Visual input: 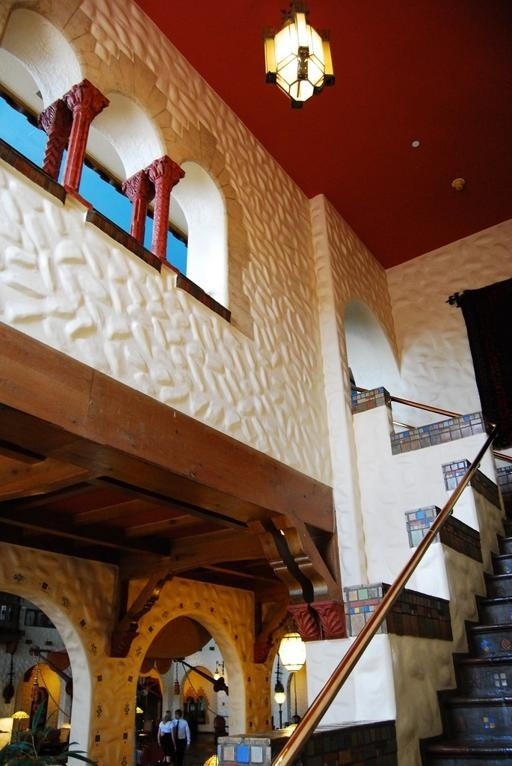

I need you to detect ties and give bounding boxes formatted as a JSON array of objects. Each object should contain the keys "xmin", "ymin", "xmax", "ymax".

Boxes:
[{"xmin": 175, "ymin": 720, "xmax": 180, "ymax": 739}]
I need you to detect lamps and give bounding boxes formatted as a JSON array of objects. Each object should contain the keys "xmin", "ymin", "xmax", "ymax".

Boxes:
[
  {"xmin": 260, "ymin": 2, "xmax": 337, "ymax": 111},
  {"xmin": 275, "ymin": 631, "xmax": 308, "ymax": 728}
]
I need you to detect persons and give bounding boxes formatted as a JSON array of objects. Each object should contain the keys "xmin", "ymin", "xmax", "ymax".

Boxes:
[
  {"xmin": 157, "ymin": 711, "xmax": 177, "ymax": 766},
  {"xmin": 170, "ymin": 710, "xmax": 193, "ymax": 766},
  {"xmin": 288, "ymin": 715, "xmax": 301, "ymax": 729}
]
